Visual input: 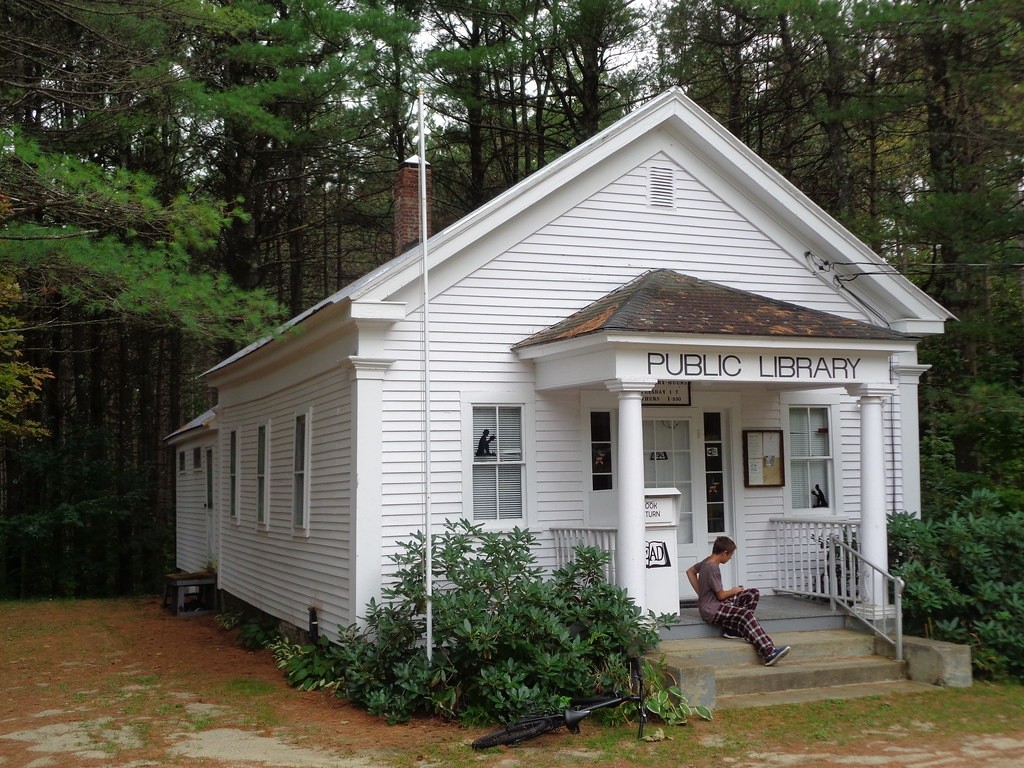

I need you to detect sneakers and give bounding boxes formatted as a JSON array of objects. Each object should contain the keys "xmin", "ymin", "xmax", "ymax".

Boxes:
[
  {"xmin": 764, "ymin": 645, "xmax": 791, "ymax": 665},
  {"xmin": 723, "ymin": 628, "xmax": 743, "ymax": 639}
]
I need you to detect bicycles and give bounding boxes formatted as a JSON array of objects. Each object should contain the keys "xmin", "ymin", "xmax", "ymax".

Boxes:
[{"xmin": 471, "ymin": 656, "xmax": 653, "ymax": 748}]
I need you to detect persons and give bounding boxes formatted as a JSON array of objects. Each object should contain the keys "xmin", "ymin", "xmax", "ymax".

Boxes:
[{"xmin": 685, "ymin": 535, "xmax": 791, "ymax": 667}]
